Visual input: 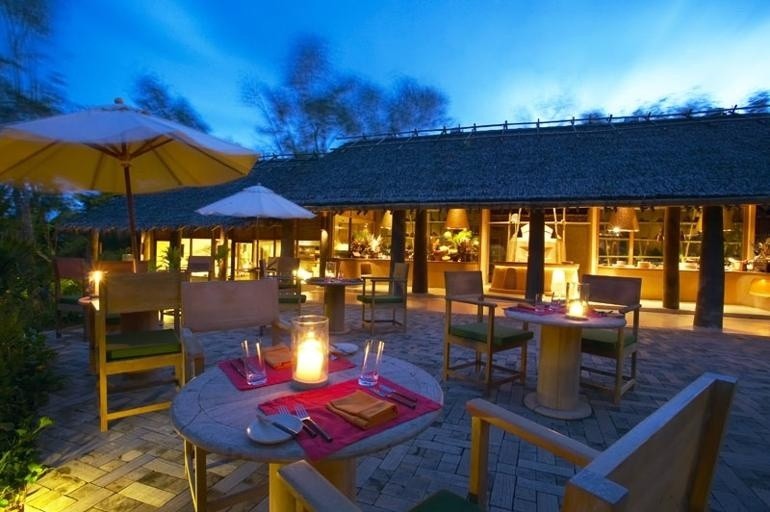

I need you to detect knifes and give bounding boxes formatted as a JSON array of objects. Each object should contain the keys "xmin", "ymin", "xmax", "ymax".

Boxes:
[
  {"xmin": 231, "ymin": 356, "xmax": 260, "ymax": 380},
  {"xmin": 378, "ymin": 383, "xmax": 418, "ymax": 402},
  {"xmin": 367, "ymin": 385, "xmax": 416, "ymax": 409},
  {"xmin": 256, "ymin": 413, "xmax": 298, "ymax": 437},
  {"xmin": 330, "ymin": 343, "xmax": 352, "ymax": 354}
]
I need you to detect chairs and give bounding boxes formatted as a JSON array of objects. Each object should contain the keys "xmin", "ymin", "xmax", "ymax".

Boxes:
[
  {"xmin": 356, "ymin": 262, "xmax": 410, "ymax": 337},
  {"xmin": 53, "ymin": 257, "xmax": 90, "ymax": 342},
  {"xmin": 275, "ymin": 372, "xmax": 738, "ymax": 512},
  {"xmin": 579, "ymin": 274, "xmax": 642, "ymax": 405},
  {"xmin": 267, "ymin": 257, "xmax": 301, "ymax": 316},
  {"xmin": 95, "ymin": 260, "xmax": 150, "ymax": 297},
  {"xmin": 443, "ymin": 271, "xmax": 533, "ymax": 397},
  {"xmin": 94, "ymin": 268, "xmax": 188, "ymax": 431},
  {"xmin": 186, "ymin": 256, "xmax": 216, "ymax": 283}
]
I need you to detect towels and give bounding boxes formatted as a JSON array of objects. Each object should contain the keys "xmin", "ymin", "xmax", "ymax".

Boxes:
[
  {"xmin": 262, "ymin": 342, "xmax": 291, "ymax": 370},
  {"xmin": 324, "ymin": 390, "xmax": 398, "ymax": 430}
]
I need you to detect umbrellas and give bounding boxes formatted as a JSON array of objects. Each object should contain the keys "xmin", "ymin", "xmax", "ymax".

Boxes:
[
  {"xmin": 193, "ymin": 182, "xmax": 316, "ymax": 268},
  {"xmin": 0, "ymin": 98, "xmax": 261, "ymax": 275}
]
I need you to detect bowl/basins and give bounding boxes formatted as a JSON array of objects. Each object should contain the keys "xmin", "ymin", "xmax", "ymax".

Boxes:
[{"xmin": 638, "ymin": 262, "xmax": 651, "ymax": 269}]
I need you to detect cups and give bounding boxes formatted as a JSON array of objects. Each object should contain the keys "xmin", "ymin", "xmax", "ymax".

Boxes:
[
  {"xmin": 566, "ymin": 282, "xmax": 590, "ymax": 319},
  {"xmin": 552, "ymin": 288, "xmax": 562, "ymax": 311},
  {"xmin": 290, "ymin": 315, "xmax": 329, "ymax": 390},
  {"xmin": 534, "ymin": 293, "xmax": 546, "ymax": 315},
  {"xmin": 358, "ymin": 338, "xmax": 385, "ymax": 386},
  {"xmin": 616, "ymin": 261, "xmax": 625, "ymax": 267},
  {"xmin": 240, "ymin": 337, "xmax": 267, "ymax": 385}
]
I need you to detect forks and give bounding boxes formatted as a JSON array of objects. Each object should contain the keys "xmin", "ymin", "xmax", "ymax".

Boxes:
[
  {"xmin": 278, "ymin": 405, "xmax": 316, "ymax": 438},
  {"xmin": 329, "ymin": 352, "xmax": 339, "ymax": 361},
  {"xmin": 294, "ymin": 403, "xmax": 332, "ymax": 441}
]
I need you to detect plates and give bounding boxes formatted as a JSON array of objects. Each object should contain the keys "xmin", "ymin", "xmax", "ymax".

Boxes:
[
  {"xmin": 245, "ymin": 414, "xmax": 304, "ymax": 445},
  {"xmin": 329, "ymin": 343, "xmax": 358, "ymax": 356}
]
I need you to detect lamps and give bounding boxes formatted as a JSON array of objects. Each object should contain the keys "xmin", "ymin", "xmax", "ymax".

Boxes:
[
  {"xmin": 378, "ymin": 209, "xmax": 393, "ymax": 229},
  {"xmin": 607, "ymin": 206, "xmax": 641, "ymax": 233},
  {"xmin": 442, "ymin": 209, "xmax": 470, "ymax": 229}
]
